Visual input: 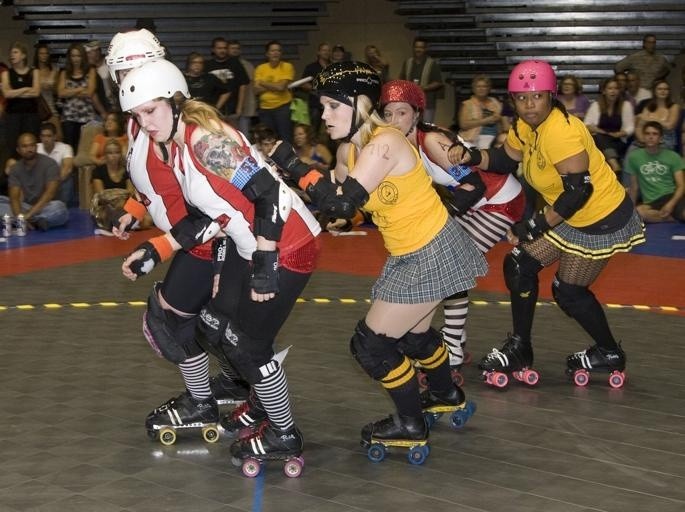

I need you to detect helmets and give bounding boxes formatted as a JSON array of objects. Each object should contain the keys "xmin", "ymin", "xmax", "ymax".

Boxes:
[
  {"xmin": 507, "ymin": 61, "xmax": 558, "ymax": 93},
  {"xmin": 313, "ymin": 60, "xmax": 382, "ymax": 108},
  {"xmin": 104, "ymin": 27, "xmax": 167, "ymax": 85},
  {"xmin": 381, "ymin": 79, "xmax": 426, "ymax": 109},
  {"xmin": 118, "ymin": 58, "xmax": 192, "ymax": 114}
]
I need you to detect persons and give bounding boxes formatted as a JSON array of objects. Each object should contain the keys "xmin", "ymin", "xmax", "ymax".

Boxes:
[
  {"xmin": 2, "ymin": 15, "xmax": 685, "ymax": 240},
  {"xmin": 120, "ymin": 59, "xmax": 323, "ymax": 478},
  {"xmin": 446, "ymin": 60, "xmax": 649, "ymax": 389},
  {"xmin": 104, "ymin": 28, "xmax": 251, "ymax": 447},
  {"xmin": 380, "ymin": 80, "xmax": 528, "ymax": 393},
  {"xmin": 267, "ymin": 59, "xmax": 489, "ymax": 467}
]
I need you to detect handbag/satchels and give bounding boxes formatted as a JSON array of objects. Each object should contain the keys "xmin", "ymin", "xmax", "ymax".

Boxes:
[{"xmin": 36, "ymin": 95, "xmax": 52, "ymax": 121}]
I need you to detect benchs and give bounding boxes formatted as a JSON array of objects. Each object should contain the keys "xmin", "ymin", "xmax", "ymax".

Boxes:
[{"xmin": 393, "ymin": 0, "xmax": 685, "ymax": 133}]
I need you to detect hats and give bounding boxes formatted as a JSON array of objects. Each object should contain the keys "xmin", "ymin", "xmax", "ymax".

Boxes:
[{"xmin": 333, "ymin": 44, "xmax": 344, "ymax": 52}]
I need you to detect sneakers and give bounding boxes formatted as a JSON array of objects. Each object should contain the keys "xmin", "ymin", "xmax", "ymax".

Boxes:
[{"xmin": 30, "ymin": 219, "xmax": 48, "ymax": 232}]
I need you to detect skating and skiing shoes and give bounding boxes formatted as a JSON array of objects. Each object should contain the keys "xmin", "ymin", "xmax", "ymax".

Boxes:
[
  {"xmin": 419, "ymin": 385, "xmax": 476, "ymax": 431},
  {"xmin": 144, "ymin": 370, "xmax": 304, "ymax": 478},
  {"xmin": 416, "ymin": 340, "xmax": 626, "ymax": 388},
  {"xmin": 360, "ymin": 412, "xmax": 430, "ymax": 464}
]
